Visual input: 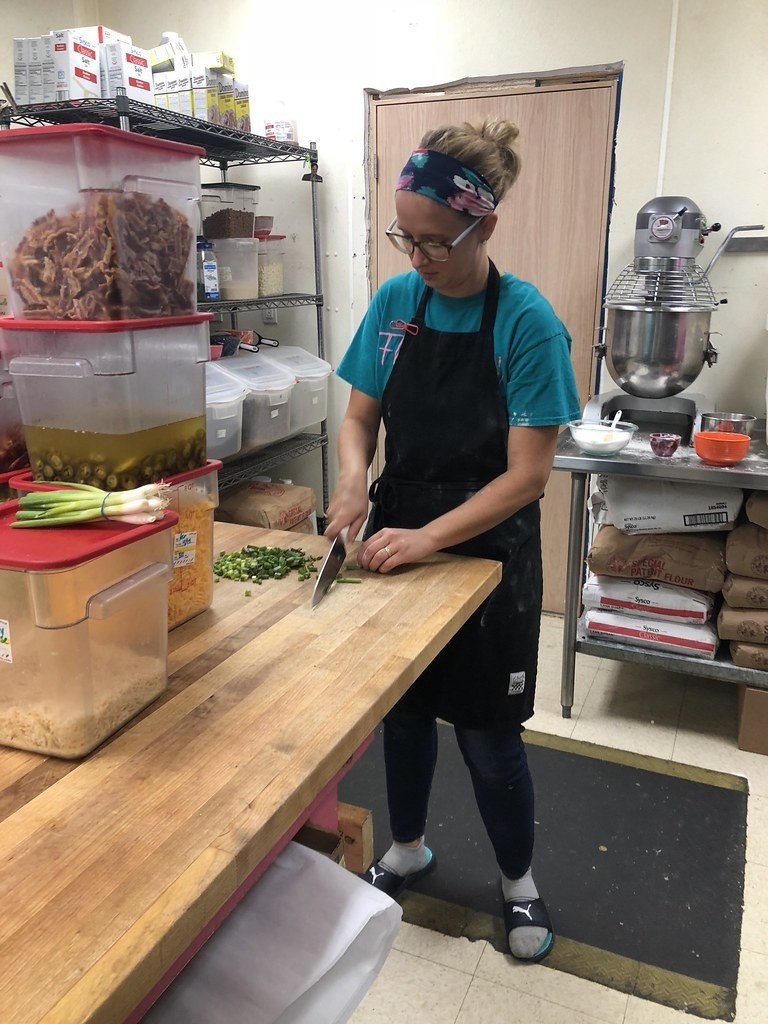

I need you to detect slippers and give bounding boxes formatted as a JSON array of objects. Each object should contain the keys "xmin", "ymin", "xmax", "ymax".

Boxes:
[
  {"xmin": 499, "ymin": 877, "xmax": 554, "ymax": 962},
  {"xmin": 364, "ymin": 856, "xmax": 437, "ymax": 900}
]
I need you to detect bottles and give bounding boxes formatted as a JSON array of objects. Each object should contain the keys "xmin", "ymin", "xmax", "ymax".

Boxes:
[{"xmin": 196, "ymin": 243, "xmax": 221, "ymax": 301}]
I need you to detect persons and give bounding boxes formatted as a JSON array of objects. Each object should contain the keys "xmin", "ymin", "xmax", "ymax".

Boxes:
[{"xmin": 323, "ymin": 118, "xmax": 583, "ymax": 964}]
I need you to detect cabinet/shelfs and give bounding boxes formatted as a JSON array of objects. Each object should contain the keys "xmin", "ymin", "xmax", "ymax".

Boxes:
[
  {"xmin": 550, "ymin": 416, "xmax": 768, "ymax": 720},
  {"xmin": 0, "ymin": 86, "xmax": 331, "ymax": 536}
]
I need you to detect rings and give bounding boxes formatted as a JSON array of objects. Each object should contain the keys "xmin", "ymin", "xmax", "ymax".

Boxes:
[{"xmin": 384, "ymin": 547, "xmax": 392, "ymax": 558}]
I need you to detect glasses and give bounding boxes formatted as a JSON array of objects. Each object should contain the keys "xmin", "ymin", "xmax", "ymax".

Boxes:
[{"xmin": 386, "ymin": 217, "xmax": 482, "ymax": 264}]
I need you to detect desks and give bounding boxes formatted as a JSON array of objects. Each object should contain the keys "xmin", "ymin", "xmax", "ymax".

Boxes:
[{"xmin": 0, "ymin": 522, "xmax": 502, "ymax": 1023}]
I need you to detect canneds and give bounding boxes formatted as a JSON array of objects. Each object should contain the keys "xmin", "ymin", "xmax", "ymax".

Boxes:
[{"xmin": 196, "ymin": 242, "xmax": 221, "ymax": 303}]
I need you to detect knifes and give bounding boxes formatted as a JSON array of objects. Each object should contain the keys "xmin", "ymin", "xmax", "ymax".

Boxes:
[{"xmin": 310, "ymin": 525, "xmax": 349, "ymax": 607}]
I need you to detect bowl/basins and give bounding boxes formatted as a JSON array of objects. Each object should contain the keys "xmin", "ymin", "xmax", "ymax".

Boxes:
[
  {"xmin": 694, "ymin": 431, "xmax": 751, "ymax": 466},
  {"xmin": 649, "ymin": 433, "xmax": 682, "ymax": 456},
  {"xmin": 701, "ymin": 413, "xmax": 757, "ymax": 435},
  {"xmin": 567, "ymin": 420, "xmax": 638, "ymax": 456}
]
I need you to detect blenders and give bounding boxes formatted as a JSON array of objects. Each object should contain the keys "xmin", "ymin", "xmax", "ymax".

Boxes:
[{"xmin": 602, "ymin": 195, "xmax": 717, "ymax": 441}]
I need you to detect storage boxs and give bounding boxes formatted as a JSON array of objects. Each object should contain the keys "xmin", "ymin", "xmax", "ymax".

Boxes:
[
  {"xmin": 737, "ymin": 685, "xmax": 768, "ymax": 755},
  {"xmin": 198, "ymin": 183, "xmax": 261, "ymax": 241},
  {"xmin": 202, "ymin": 238, "xmax": 260, "ymax": 301},
  {"xmin": 8, "ymin": 458, "xmax": 223, "ymax": 634},
  {"xmin": 0, "ymin": 314, "xmax": 216, "ymax": 494},
  {"xmin": 254, "ymin": 235, "xmax": 286, "ymax": 299},
  {"xmin": 12, "ymin": 24, "xmax": 251, "ymax": 135},
  {"xmin": 1, "ymin": 123, "xmax": 207, "ymax": 321},
  {"xmin": 203, "ymin": 362, "xmax": 252, "ymax": 459},
  {"xmin": 1, "ymin": 497, "xmax": 180, "ymax": 762},
  {"xmin": 258, "ymin": 344, "xmax": 332, "ymax": 429},
  {"xmin": 337, "ymin": 801, "xmax": 374, "ymax": 875},
  {"xmin": 214, "ymin": 352, "xmax": 300, "ymax": 449}
]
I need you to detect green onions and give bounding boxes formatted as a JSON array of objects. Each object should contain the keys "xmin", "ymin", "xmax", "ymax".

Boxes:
[
  {"xmin": 213, "ymin": 544, "xmax": 364, "ymax": 596},
  {"xmin": 9, "ymin": 480, "xmax": 172, "ymax": 524}
]
